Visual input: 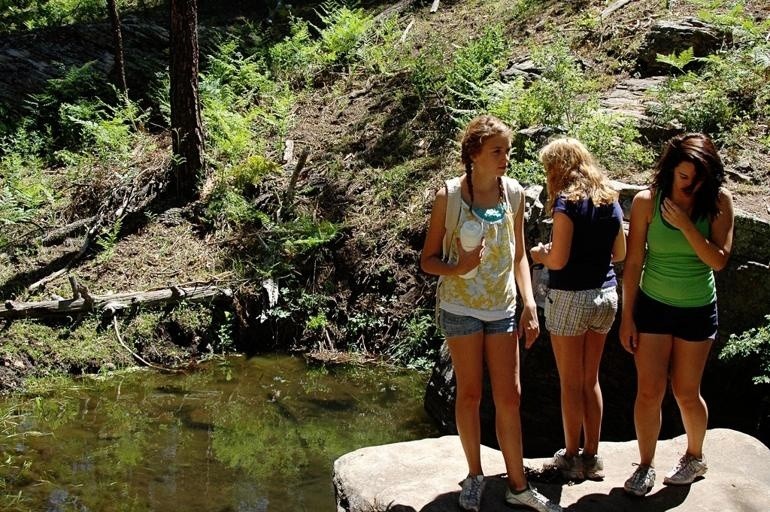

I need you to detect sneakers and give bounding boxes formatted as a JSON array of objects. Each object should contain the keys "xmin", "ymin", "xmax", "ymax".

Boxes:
[
  {"xmin": 623, "ymin": 463, "xmax": 658, "ymax": 499},
  {"xmin": 661, "ymin": 452, "xmax": 709, "ymax": 488},
  {"xmin": 457, "ymin": 472, "xmax": 488, "ymax": 509},
  {"xmin": 502, "ymin": 481, "xmax": 563, "ymax": 512},
  {"xmin": 554, "ymin": 445, "xmax": 607, "ymax": 485}
]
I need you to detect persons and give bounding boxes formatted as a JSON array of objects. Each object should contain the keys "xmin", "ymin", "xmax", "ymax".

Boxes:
[
  {"xmin": 616, "ymin": 133, "xmax": 736, "ymax": 499},
  {"xmin": 419, "ymin": 113, "xmax": 569, "ymax": 512},
  {"xmin": 527, "ymin": 136, "xmax": 629, "ymax": 486}
]
